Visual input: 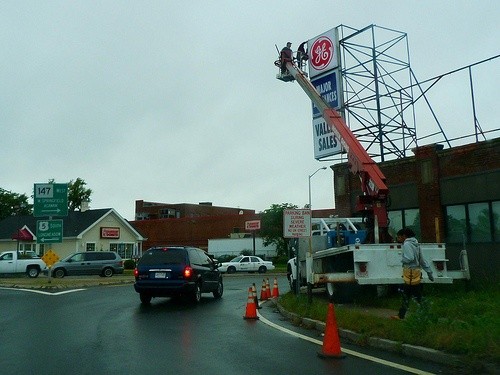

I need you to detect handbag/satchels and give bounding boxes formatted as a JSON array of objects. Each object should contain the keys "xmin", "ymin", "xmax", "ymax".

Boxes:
[{"xmin": 403, "ymin": 267, "xmax": 422, "ymax": 286}]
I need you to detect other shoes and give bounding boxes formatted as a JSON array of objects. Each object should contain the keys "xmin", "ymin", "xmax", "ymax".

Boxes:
[{"xmin": 390, "ymin": 315, "xmax": 400, "ymax": 319}]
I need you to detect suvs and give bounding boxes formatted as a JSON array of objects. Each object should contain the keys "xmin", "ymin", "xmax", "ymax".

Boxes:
[{"xmin": 134, "ymin": 246, "xmax": 223, "ymax": 305}]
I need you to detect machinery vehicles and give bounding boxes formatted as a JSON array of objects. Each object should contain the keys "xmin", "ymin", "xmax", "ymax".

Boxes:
[{"xmin": 275, "ymin": 44, "xmax": 471, "ymax": 297}]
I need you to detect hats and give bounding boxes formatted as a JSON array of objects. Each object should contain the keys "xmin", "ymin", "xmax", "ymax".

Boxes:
[{"xmin": 287, "ymin": 42, "xmax": 292, "ymax": 44}]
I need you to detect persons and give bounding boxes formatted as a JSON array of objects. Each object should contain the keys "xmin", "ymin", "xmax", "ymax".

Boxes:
[
  {"xmin": 279, "ymin": 42, "xmax": 292, "ymax": 75},
  {"xmin": 296, "ymin": 41, "xmax": 308, "ymax": 68},
  {"xmin": 390, "ymin": 228, "xmax": 433, "ymax": 321}
]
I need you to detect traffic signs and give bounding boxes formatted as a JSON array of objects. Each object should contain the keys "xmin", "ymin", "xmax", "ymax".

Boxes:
[
  {"xmin": 34, "ymin": 183, "xmax": 68, "ymax": 218},
  {"xmin": 36, "ymin": 219, "xmax": 63, "ymax": 244}
]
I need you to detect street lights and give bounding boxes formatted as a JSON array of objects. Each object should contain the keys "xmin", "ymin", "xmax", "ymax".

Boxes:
[
  {"xmin": 306, "ymin": 166, "xmax": 327, "ymax": 303},
  {"xmin": 14, "ymin": 205, "xmax": 21, "ymax": 250}
]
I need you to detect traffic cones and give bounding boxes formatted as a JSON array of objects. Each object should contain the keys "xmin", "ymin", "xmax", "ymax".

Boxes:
[
  {"xmin": 315, "ymin": 302, "xmax": 347, "ymax": 359},
  {"xmin": 242, "ymin": 283, "xmax": 262, "ymax": 320},
  {"xmin": 258, "ymin": 277, "xmax": 273, "ymax": 301},
  {"xmin": 270, "ymin": 277, "xmax": 279, "ymax": 299}
]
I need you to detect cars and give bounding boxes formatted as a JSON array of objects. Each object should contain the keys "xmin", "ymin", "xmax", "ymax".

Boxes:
[
  {"xmin": 213, "ymin": 255, "xmax": 275, "ymax": 274},
  {"xmin": 0, "ymin": 250, "xmax": 47, "ymax": 278}
]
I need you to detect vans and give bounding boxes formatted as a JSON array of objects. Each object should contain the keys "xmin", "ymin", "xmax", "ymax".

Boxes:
[{"xmin": 44, "ymin": 251, "xmax": 125, "ymax": 279}]
